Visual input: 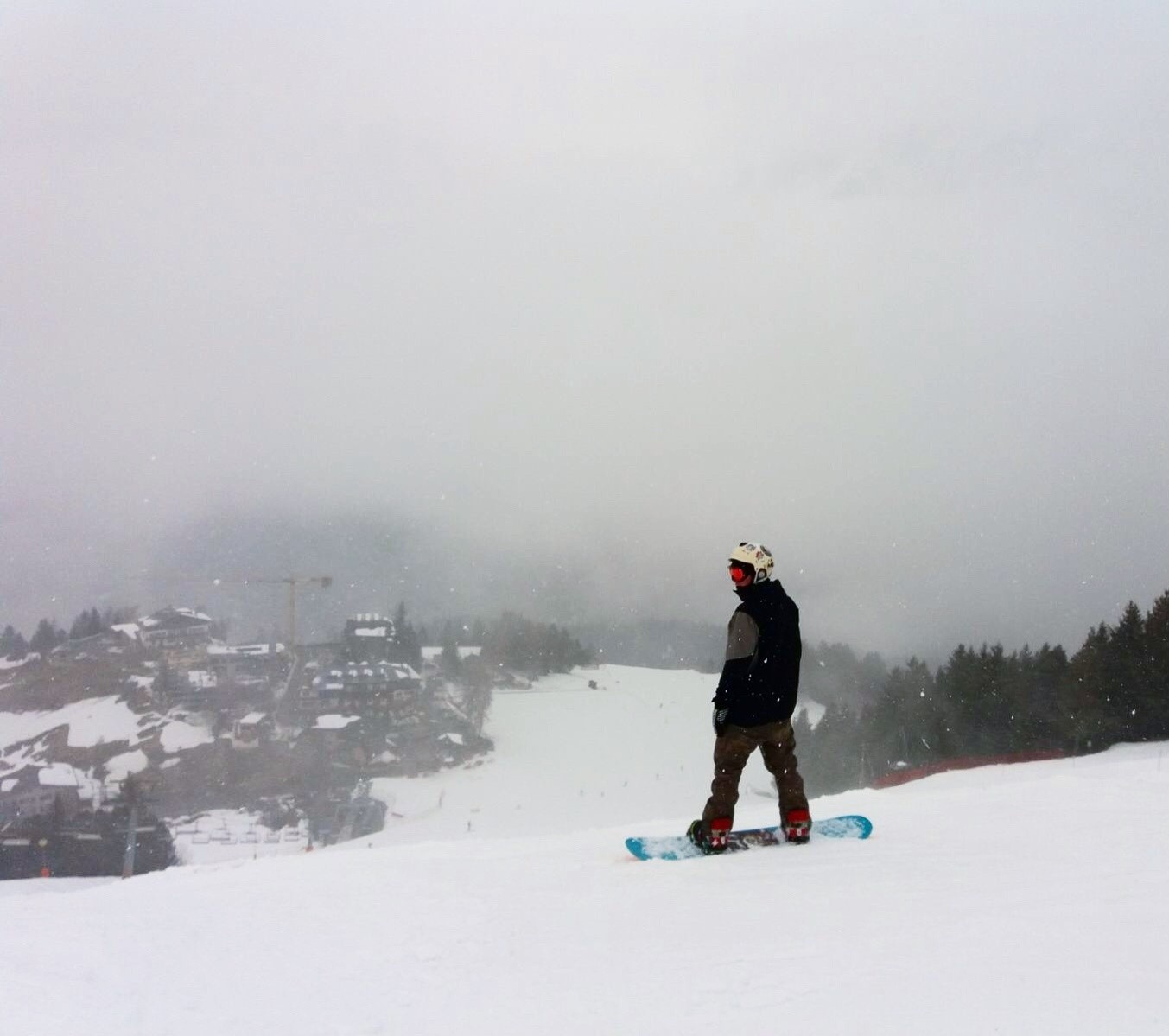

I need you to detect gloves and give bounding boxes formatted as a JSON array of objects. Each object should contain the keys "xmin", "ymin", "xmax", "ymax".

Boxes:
[{"xmin": 710, "ymin": 691, "xmax": 732, "ymax": 738}]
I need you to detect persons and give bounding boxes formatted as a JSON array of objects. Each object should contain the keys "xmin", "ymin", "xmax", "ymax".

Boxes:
[{"xmin": 687, "ymin": 542, "xmax": 812, "ymax": 856}]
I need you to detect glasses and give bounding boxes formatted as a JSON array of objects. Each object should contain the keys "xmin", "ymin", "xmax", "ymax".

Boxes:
[{"xmin": 728, "ymin": 565, "xmax": 755, "ymax": 582}]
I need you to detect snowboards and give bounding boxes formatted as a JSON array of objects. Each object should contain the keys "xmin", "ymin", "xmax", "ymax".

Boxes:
[{"xmin": 625, "ymin": 815, "xmax": 873, "ymax": 861}]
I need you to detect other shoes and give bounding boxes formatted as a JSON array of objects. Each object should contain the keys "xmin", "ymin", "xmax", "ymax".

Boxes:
[
  {"xmin": 786, "ymin": 828, "xmax": 813, "ymax": 846},
  {"xmin": 690, "ymin": 821, "xmax": 729, "ymax": 857}
]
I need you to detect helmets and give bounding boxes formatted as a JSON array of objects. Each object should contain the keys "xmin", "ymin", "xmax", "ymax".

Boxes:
[{"xmin": 726, "ymin": 542, "xmax": 776, "ymax": 585}]
